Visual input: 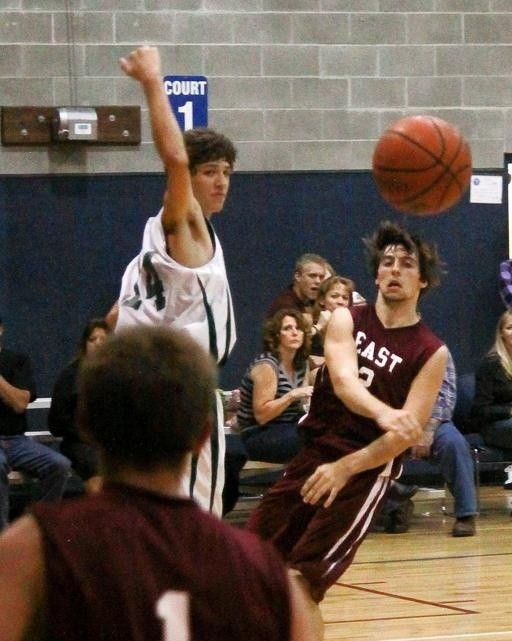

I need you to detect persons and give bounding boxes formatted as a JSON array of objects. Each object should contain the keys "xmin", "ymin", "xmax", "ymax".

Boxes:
[
  {"xmin": 240, "ymin": 219, "xmax": 444, "ymax": 602},
  {"xmin": 0, "ymin": 318, "xmax": 113, "ymax": 507},
  {"xmin": 104, "ymin": 38, "xmax": 239, "ymax": 518},
  {"xmin": 1, "ymin": 322, "xmax": 327, "ymax": 641},
  {"xmin": 219, "ymin": 249, "xmax": 511, "ymax": 542}
]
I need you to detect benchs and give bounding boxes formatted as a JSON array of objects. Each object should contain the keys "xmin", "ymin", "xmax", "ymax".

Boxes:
[{"xmin": 8, "ymin": 392, "xmax": 283, "ymax": 487}]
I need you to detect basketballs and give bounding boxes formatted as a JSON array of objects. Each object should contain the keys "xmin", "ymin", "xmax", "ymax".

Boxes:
[{"xmin": 371, "ymin": 114, "xmax": 473, "ymax": 219}]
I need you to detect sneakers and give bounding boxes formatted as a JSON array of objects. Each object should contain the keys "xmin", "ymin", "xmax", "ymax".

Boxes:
[
  {"xmin": 453, "ymin": 516, "xmax": 474, "ymax": 536},
  {"xmin": 387, "ymin": 500, "xmax": 413, "ymax": 533}
]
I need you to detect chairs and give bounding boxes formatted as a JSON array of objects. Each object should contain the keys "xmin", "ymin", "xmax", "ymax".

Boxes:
[{"xmin": 442, "ymin": 373, "xmax": 512, "ymax": 517}]
